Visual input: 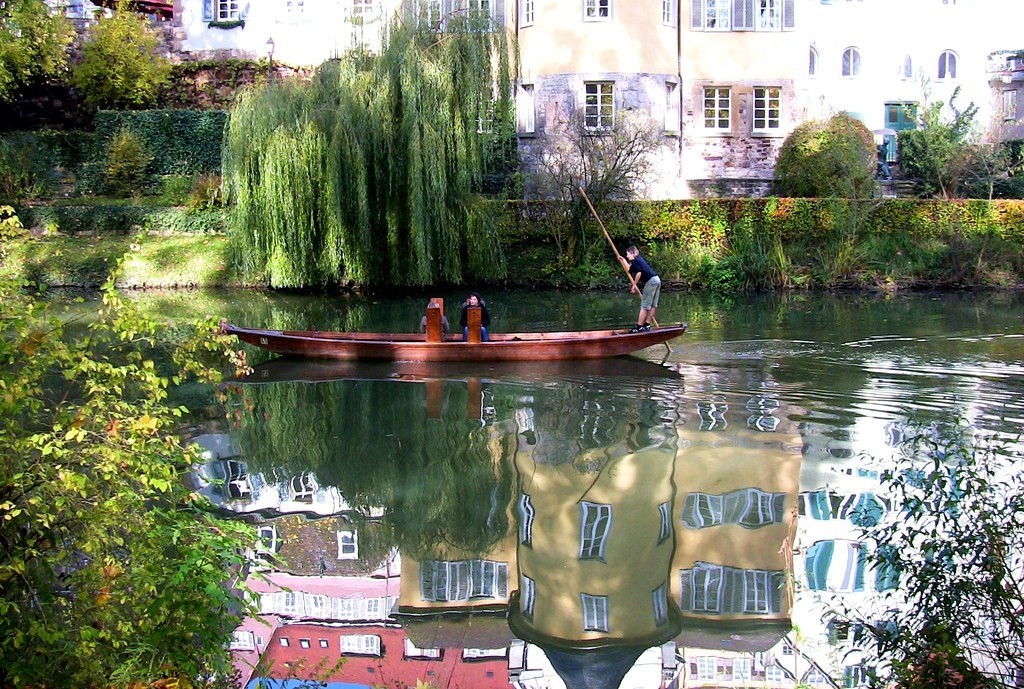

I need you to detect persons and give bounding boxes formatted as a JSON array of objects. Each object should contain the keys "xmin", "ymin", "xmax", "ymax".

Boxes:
[
  {"xmin": 420, "ymin": 302, "xmax": 449, "ymax": 343},
  {"xmin": 876, "ymin": 138, "xmax": 893, "ymax": 180},
  {"xmin": 460, "ymin": 292, "xmax": 491, "ymax": 342},
  {"xmin": 618, "ymin": 246, "xmax": 661, "ymax": 335}
]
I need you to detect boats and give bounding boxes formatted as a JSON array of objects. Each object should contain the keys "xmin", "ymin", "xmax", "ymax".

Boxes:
[
  {"xmin": 221, "ymin": 361, "xmax": 685, "ymax": 420},
  {"xmin": 206, "ymin": 297, "xmax": 688, "ymax": 361}
]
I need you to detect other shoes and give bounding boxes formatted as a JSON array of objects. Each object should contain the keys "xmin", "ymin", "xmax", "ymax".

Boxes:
[
  {"xmin": 643, "ymin": 324, "xmax": 650, "ymax": 331},
  {"xmin": 629, "ymin": 327, "xmax": 643, "ymax": 333}
]
